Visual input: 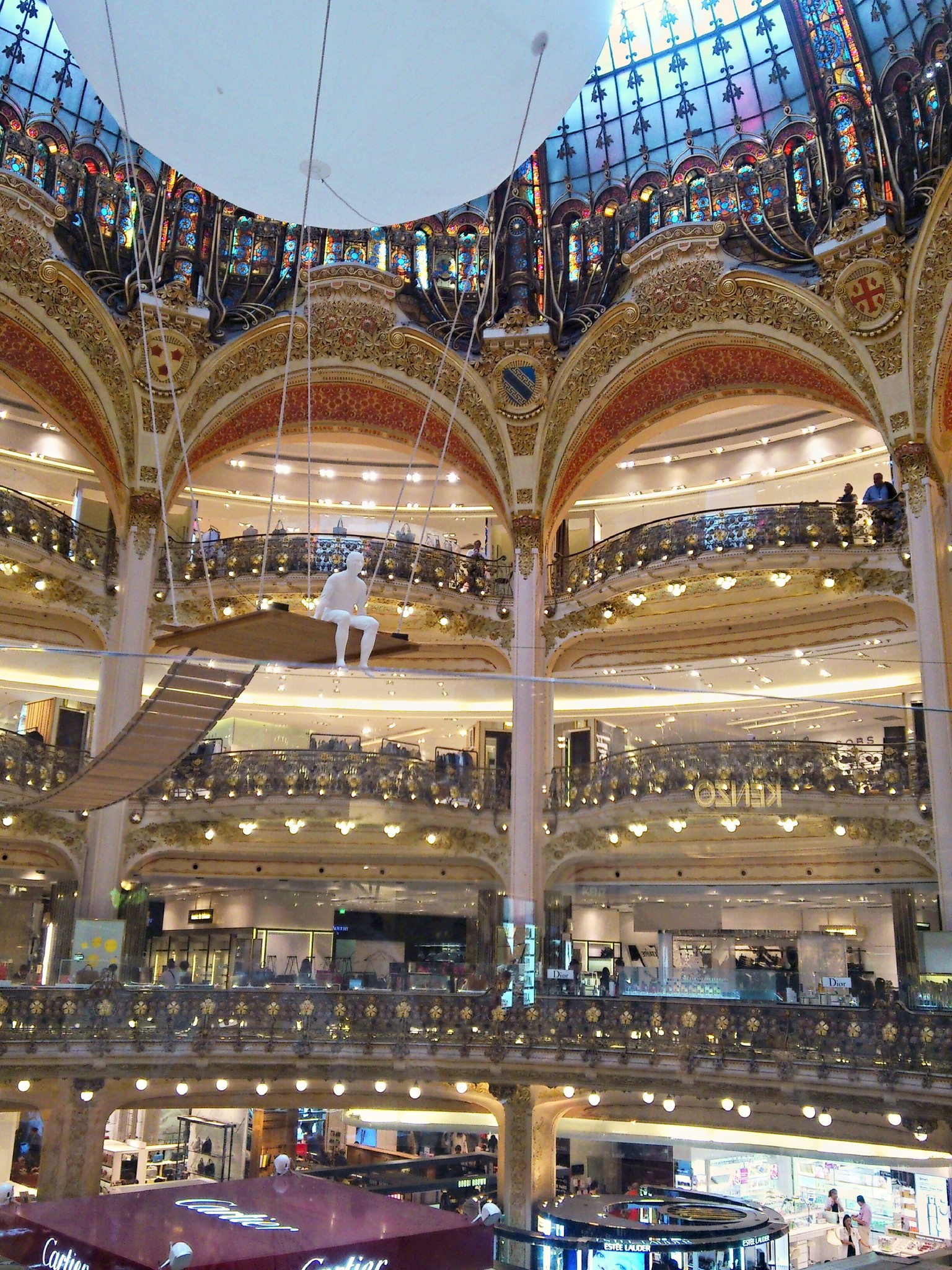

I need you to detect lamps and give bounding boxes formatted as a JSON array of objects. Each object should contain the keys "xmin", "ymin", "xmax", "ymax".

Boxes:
[{"xmin": 0, "ymin": 539, "xmax": 927, "ymax": 1141}]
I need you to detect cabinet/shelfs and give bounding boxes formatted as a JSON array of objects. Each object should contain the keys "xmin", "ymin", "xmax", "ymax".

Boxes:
[
  {"xmin": 573, "ymin": 940, "xmax": 622, "ymax": 974},
  {"xmin": 101, "ymin": 1115, "xmax": 236, "ymax": 1186},
  {"xmin": 156, "ymin": 965, "xmax": 228, "ymax": 977},
  {"xmin": 705, "ymin": 1155, "xmax": 919, "ymax": 1270}
]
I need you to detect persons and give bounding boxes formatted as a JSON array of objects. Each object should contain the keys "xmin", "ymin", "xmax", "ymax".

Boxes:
[
  {"xmin": 592, "ymin": 1249, "xmax": 644, "ymax": 1270},
  {"xmin": 837, "ymin": 473, "xmax": 899, "ymax": 545},
  {"xmin": 13, "ymin": 965, "xmax": 29, "ymax": 981},
  {"xmin": 466, "ymin": 540, "xmax": 487, "ymax": 594},
  {"xmin": 824, "ymin": 1189, "xmax": 872, "ymax": 1258},
  {"xmin": 311, "ymin": 552, "xmax": 380, "ymax": 677},
  {"xmin": 333, "ymin": 1148, "xmax": 347, "ymax": 1183},
  {"xmin": 18, "ymin": 1128, "xmax": 41, "ymax": 1174},
  {"xmin": 465, "ymin": 964, "xmax": 487, "ymax": 991},
  {"xmin": 159, "ymin": 958, "xmax": 192, "ymax": 987},
  {"xmin": 588, "ymin": 1180, "xmax": 641, "ymax": 1221},
  {"xmin": 565, "ymin": 958, "xmax": 629, "ymax": 998}
]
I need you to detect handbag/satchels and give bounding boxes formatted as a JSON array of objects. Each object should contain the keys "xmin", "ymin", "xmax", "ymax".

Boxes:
[
  {"xmin": 76, "ymin": 965, "xmax": 98, "ymax": 984},
  {"xmin": 146, "ymin": 1167, "xmax": 158, "ymax": 1179},
  {"xmin": 250, "ymin": 958, "xmax": 342, "ymax": 986},
  {"xmin": 202, "ymin": 1137, "xmax": 213, "ymax": 1153},
  {"xmin": 197, "ymin": 1158, "xmax": 205, "ymax": 1174},
  {"xmin": 272, "ymin": 521, "xmax": 287, "ymax": 539},
  {"xmin": 172, "ymin": 1148, "xmax": 184, "ymax": 1160},
  {"xmin": 163, "ymin": 1168, "xmax": 173, "ymax": 1177},
  {"xmin": 152, "ymin": 1151, "xmax": 164, "ymax": 1163},
  {"xmin": 395, "ymin": 523, "xmax": 415, "ymax": 547},
  {"xmin": 190, "ymin": 1137, "xmax": 201, "ymax": 1151},
  {"xmin": 204, "ymin": 1159, "xmax": 215, "ymax": 1175},
  {"xmin": 333, "ymin": 519, "xmax": 347, "ymax": 538}
]
